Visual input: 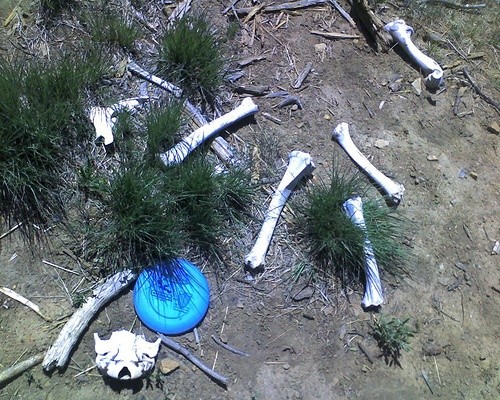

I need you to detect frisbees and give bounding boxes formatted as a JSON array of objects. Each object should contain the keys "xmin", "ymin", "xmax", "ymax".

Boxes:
[{"xmin": 133, "ymin": 258, "xmax": 210, "ymax": 336}]
